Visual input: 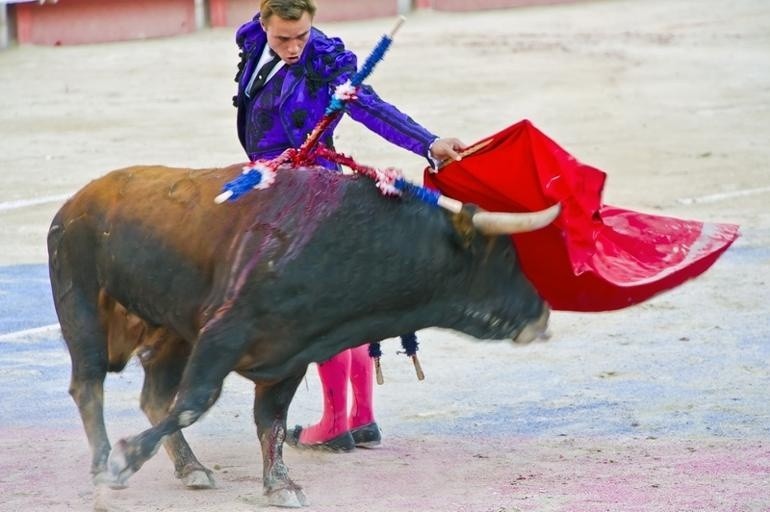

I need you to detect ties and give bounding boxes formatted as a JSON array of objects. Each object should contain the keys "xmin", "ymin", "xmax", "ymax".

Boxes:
[{"xmin": 250, "ymin": 47, "xmax": 283, "ymax": 98}]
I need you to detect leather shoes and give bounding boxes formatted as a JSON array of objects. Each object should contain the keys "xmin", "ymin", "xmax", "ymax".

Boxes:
[{"xmin": 283, "ymin": 422, "xmax": 382, "ymax": 454}]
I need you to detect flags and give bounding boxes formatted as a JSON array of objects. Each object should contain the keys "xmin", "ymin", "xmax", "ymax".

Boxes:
[{"xmin": 427, "ymin": 120, "xmax": 742, "ymax": 314}]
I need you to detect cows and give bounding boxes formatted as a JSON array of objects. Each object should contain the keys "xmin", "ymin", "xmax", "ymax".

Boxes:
[{"xmin": 45, "ymin": 160, "xmax": 561, "ymax": 509}]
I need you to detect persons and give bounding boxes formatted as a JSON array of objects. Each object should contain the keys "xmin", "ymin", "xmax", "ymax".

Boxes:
[{"xmin": 235, "ymin": 0, "xmax": 466, "ymax": 455}]
